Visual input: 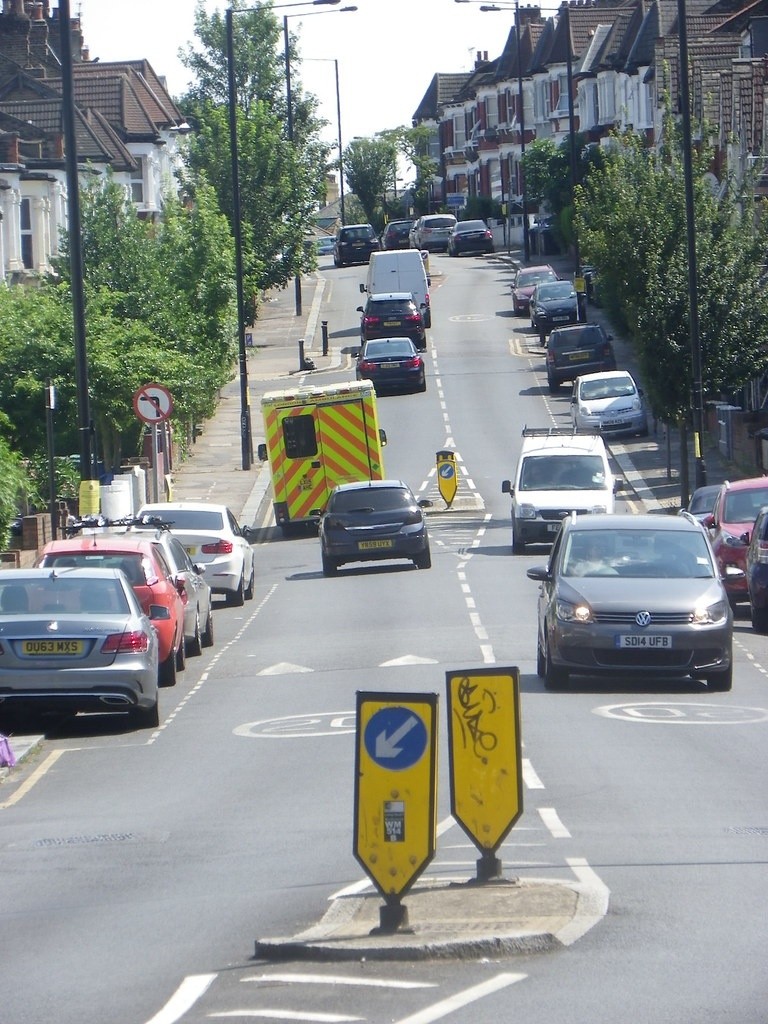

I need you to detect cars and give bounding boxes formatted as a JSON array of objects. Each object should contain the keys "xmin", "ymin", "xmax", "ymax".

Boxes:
[
  {"xmin": 447, "ymin": 220, "xmax": 495, "ymax": 257},
  {"xmin": 409, "ymin": 219, "xmax": 419, "ymax": 249},
  {"xmin": 529, "ymin": 281, "xmax": 577, "ymax": 333},
  {"xmin": 413, "ymin": 215, "xmax": 457, "ymax": 252},
  {"xmin": 351, "ymin": 337, "xmax": 428, "ymax": 393},
  {"xmin": 677, "ymin": 487, "xmax": 723, "ymax": 529},
  {"xmin": 141, "ymin": 503, "xmax": 257, "ymax": 608},
  {"xmin": 275, "ymin": 246, "xmax": 289, "ymax": 260},
  {"xmin": 31, "ymin": 536, "xmax": 189, "ymax": 687},
  {"xmin": 380, "ymin": 219, "xmax": 413, "ymax": 251},
  {"xmin": 308, "ymin": 480, "xmax": 432, "ymax": 578},
  {"xmin": 572, "ymin": 371, "xmax": 649, "ymax": 439},
  {"xmin": 0, "ymin": 567, "xmax": 171, "ymax": 730},
  {"xmin": 316, "ymin": 236, "xmax": 336, "ymax": 256},
  {"xmin": 741, "ymin": 507, "xmax": 768, "ymax": 634}
]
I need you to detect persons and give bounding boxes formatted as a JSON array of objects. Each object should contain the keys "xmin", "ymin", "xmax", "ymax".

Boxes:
[
  {"xmin": 528, "ymin": 467, "xmax": 552, "ymax": 488},
  {"xmin": 574, "ymin": 546, "xmax": 608, "ymax": 576}
]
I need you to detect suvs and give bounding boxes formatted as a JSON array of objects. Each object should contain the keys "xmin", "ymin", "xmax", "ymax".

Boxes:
[
  {"xmin": 356, "ymin": 292, "xmax": 429, "ymax": 353},
  {"xmin": 543, "ymin": 322, "xmax": 619, "ymax": 393},
  {"xmin": 703, "ymin": 473, "xmax": 767, "ymax": 600},
  {"xmin": 526, "ymin": 510, "xmax": 746, "ymax": 691},
  {"xmin": 61, "ymin": 513, "xmax": 213, "ymax": 658},
  {"xmin": 501, "ymin": 426, "xmax": 623, "ymax": 553},
  {"xmin": 330, "ymin": 223, "xmax": 380, "ymax": 268},
  {"xmin": 511, "ymin": 264, "xmax": 564, "ymax": 315}
]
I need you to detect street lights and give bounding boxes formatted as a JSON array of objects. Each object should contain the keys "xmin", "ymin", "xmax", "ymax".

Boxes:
[
  {"xmin": 453, "ymin": 0, "xmax": 531, "ymax": 264},
  {"xmin": 291, "ymin": 57, "xmax": 346, "ymax": 228},
  {"xmin": 480, "ymin": 5, "xmax": 577, "ymax": 190},
  {"xmin": 285, "ymin": 5, "xmax": 358, "ymax": 321},
  {"xmin": 225, "ymin": 0, "xmax": 342, "ymax": 474}
]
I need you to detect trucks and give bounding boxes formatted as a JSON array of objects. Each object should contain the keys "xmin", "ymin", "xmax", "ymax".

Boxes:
[
  {"xmin": 257, "ymin": 379, "xmax": 387, "ymax": 535},
  {"xmin": 358, "ymin": 250, "xmax": 433, "ymax": 330}
]
